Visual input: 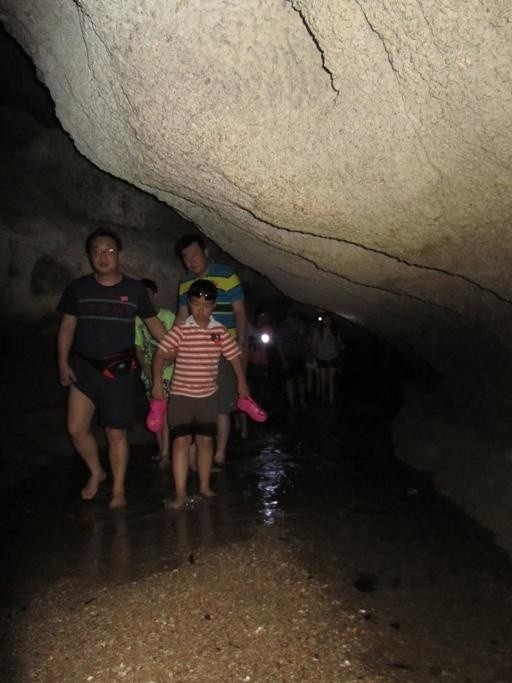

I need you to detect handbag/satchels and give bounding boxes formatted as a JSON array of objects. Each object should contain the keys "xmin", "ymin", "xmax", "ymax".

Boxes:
[{"xmin": 74, "ymin": 350, "xmax": 140, "ymax": 384}]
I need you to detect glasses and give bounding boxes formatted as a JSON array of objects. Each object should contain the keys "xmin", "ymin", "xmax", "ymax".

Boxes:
[
  {"xmin": 92, "ymin": 248, "xmax": 115, "ymax": 256},
  {"xmin": 190, "ymin": 290, "xmax": 215, "ymax": 300}
]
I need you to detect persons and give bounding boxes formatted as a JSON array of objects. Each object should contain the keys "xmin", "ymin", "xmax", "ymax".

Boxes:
[
  {"xmin": 57, "ymin": 228, "xmax": 168, "ymax": 506},
  {"xmin": 251, "ymin": 311, "xmax": 339, "ymax": 407},
  {"xmin": 153, "ymin": 280, "xmax": 251, "ymax": 507},
  {"xmin": 134, "ymin": 278, "xmax": 176, "ymax": 461},
  {"xmin": 171, "ymin": 231, "xmax": 247, "ymax": 464}
]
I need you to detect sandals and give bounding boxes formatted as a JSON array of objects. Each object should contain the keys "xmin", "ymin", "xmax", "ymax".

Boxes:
[
  {"xmin": 146, "ymin": 399, "xmax": 167, "ymax": 432},
  {"xmin": 237, "ymin": 397, "xmax": 268, "ymax": 423}
]
